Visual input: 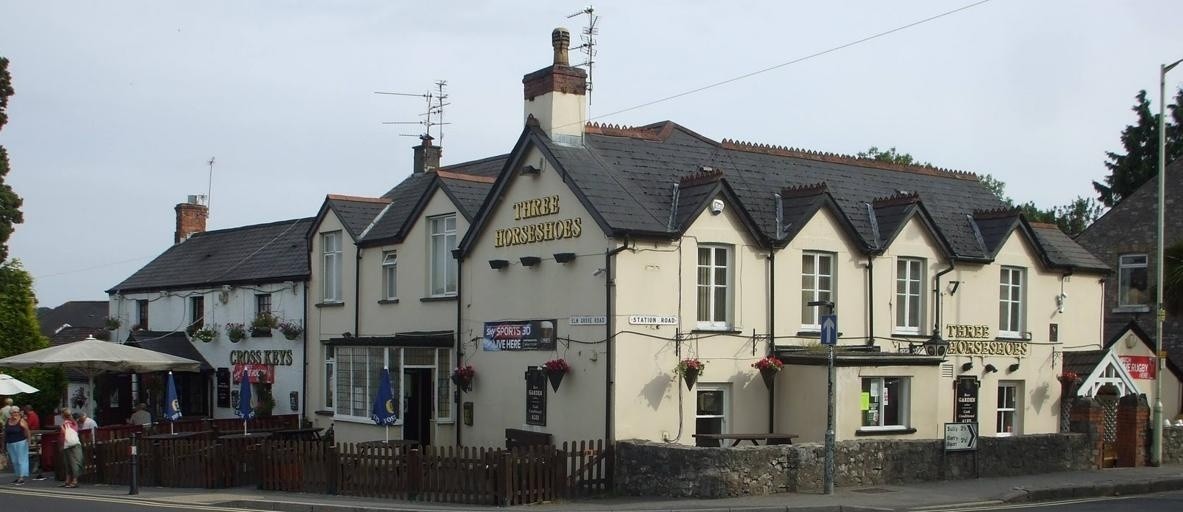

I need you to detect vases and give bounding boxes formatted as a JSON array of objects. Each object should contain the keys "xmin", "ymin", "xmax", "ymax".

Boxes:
[
  {"xmin": 547, "ymin": 369, "xmax": 566, "ymax": 393},
  {"xmin": 760, "ymin": 367, "xmax": 781, "ymax": 390},
  {"xmin": 682, "ymin": 368, "xmax": 698, "ymax": 391}
]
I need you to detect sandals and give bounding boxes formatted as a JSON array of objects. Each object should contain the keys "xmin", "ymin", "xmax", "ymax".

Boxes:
[{"xmin": 58, "ymin": 481, "xmax": 78, "ymax": 488}]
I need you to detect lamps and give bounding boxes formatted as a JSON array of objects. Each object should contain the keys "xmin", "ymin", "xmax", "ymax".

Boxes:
[
  {"xmin": 908, "ymin": 329, "xmax": 949, "ymax": 362},
  {"xmin": 521, "ymin": 165, "xmax": 541, "ymax": 177}
]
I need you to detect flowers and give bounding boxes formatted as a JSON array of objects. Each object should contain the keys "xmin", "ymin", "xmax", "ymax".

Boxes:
[
  {"xmin": 542, "ymin": 359, "xmax": 568, "ymax": 371},
  {"xmin": 751, "ymin": 354, "xmax": 784, "ymax": 371},
  {"xmin": 672, "ymin": 358, "xmax": 704, "ymax": 377}
]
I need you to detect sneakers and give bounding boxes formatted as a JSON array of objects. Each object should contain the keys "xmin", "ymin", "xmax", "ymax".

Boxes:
[{"xmin": 10, "ymin": 479, "xmax": 24, "ymax": 485}]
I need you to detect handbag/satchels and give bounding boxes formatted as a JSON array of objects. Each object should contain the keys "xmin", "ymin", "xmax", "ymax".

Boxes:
[{"xmin": 64, "ymin": 425, "xmax": 80, "ymax": 449}]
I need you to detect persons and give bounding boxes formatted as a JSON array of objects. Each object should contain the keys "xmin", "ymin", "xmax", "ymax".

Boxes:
[
  {"xmin": 72, "ymin": 411, "xmax": 98, "ymax": 429},
  {"xmin": 0, "ymin": 397, "xmax": 14, "ymax": 432},
  {"xmin": 2, "ymin": 405, "xmax": 31, "ymax": 486},
  {"xmin": 56, "ymin": 407, "xmax": 83, "ymax": 488},
  {"xmin": 22, "ymin": 404, "xmax": 40, "ymax": 430},
  {"xmin": 127, "ymin": 402, "xmax": 151, "ymax": 426}
]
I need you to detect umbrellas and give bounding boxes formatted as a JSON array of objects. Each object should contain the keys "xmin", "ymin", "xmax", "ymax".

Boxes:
[
  {"xmin": 0, "ymin": 332, "xmax": 203, "ymax": 443},
  {"xmin": 0, "ymin": 373, "xmax": 40, "ymax": 399},
  {"xmin": 162, "ymin": 370, "xmax": 183, "ymax": 435},
  {"xmin": 237, "ymin": 366, "xmax": 256, "ymax": 435},
  {"xmin": 368, "ymin": 366, "xmax": 397, "ymax": 443}
]
